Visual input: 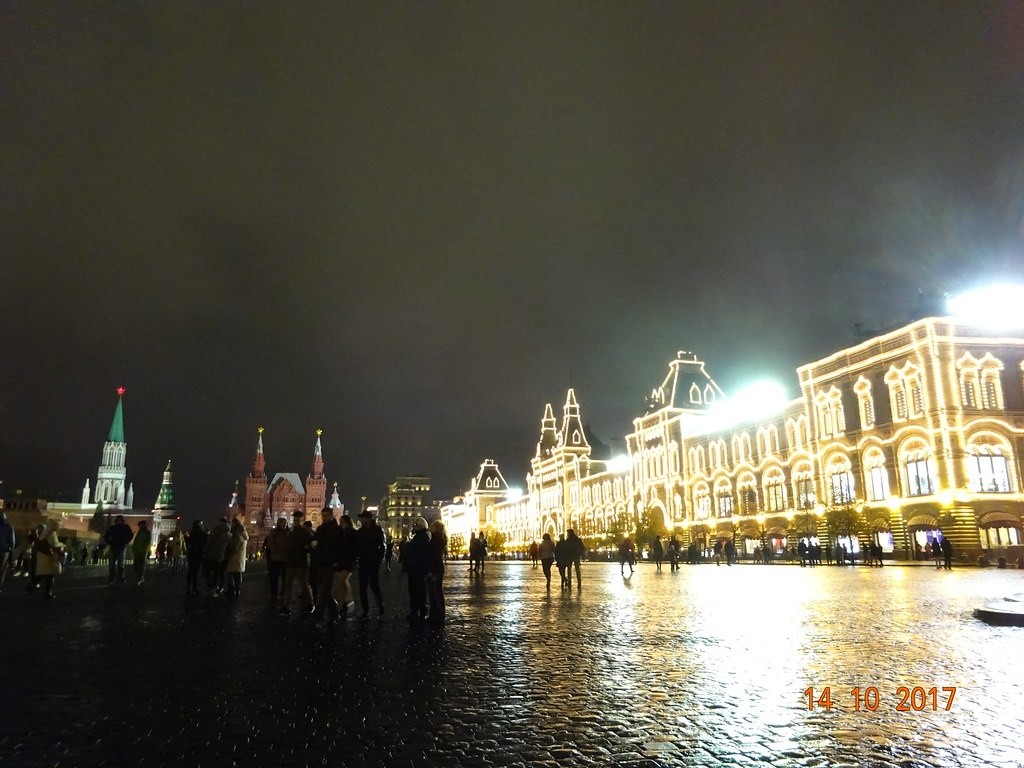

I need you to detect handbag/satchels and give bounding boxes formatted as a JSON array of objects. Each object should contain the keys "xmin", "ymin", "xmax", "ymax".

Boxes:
[{"xmin": 37, "ymin": 538, "xmax": 55, "ymax": 556}]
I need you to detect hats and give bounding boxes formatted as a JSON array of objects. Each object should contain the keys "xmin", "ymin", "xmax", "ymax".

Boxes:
[
  {"xmin": 357, "ymin": 510, "xmax": 372, "ymax": 518},
  {"xmin": 416, "ymin": 517, "xmax": 429, "ymax": 529}
]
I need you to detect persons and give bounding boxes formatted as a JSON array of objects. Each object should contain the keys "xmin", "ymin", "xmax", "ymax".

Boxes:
[
  {"xmin": 653, "ymin": 536, "xmax": 663, "ymax": 570},
  {"xmin": 132, "ymin": 521, "xmax": 151, "ymax": 588},
  {"xmin": 791, "ymin": 539, "xmax": 822, "ymax": 567},
  {"xmin": 687, "ymin": 541, "xmax": 700, "ymax": 564},
  {"xmin": 186, "ymin": 508, "xmax": 446, "ymax": 629},
  {"xmin": 619, "ymin": 538, "xmax": 635, "ymax": 573},
  {"xmin": 824, "ymin": 543, "xmax": 846, "ymax": 566},
  {"xmin": 934, "ymin": 538, "xmax": 953, "ymax": 569},
  {"xmin": 753, "ymin": 545, "xmax": 774, "ymax": 565},
  {"xmin": 468, "ymin": 532, "xmax": 487, "ymax": 573},
  {"xmin": 104, "ymin": 515, "xmax": 134, "ymax": 584},
  {"xmin": 916, "ymin": 541, "xmax": 922, "ymax": 561},
  {"xmin": 725, "ymin": 540, "xmax": 733, "ymax": 566},
  {"xmin": 862, "ymin": 541, "xmax": 884, "ymax": 568},
  {"xmin": 714, "ymin": 539, "xmax": 722, "ymax": 566},
  {"xmin": 925, "ymin": 543, "xmax": 932, "ymax": 560},
  {"xmin": 667, "ymin": 536, "xmax": 680, "ymax": 571},
  {"xmin": 528, "ymin": 530, "xmax": 584, "ymax": 589},
  {"xmin": 0, "ymin": 512, "xmax": 66, "ymax": 600}
]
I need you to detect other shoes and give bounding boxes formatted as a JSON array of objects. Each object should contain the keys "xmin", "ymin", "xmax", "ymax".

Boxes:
[
  {"xmin": 109, "ymin": 576, "xmax": 117, "ymax": 585},
  {"xmin": 135, "ymin": 578, "xmax": 145, "ymax": 586},
  {"xmin": 310, "ymin": 616, "xmax": 320, "ymax": 631},
  {"xmin": 279, "ymin": 607, "xmax": 293, "ymax": 617},
  {"xmin": 272, "ymin": 596, "xmax": 279, "ymax": 607},
  {"xmin": 192, "ymin": 589, "xmax": 201, "ymax": 595},
  {"xmin": 120, "ymin": 576, "xmax": 126, "ymax": 585},
  {"xmin": 358, "ymin": 610, "xmax": 369, "ymax": 618},
  {"xmin": 229, "ymin": 590, "xmax": 241, "ymax": 598},
  {"xmin": 48, "ymin": 592, "xmax": 56, "ymax": 600},
  {"xmin": 405, "ymin": 612, "xmax": 419, "ymax": 619}
]
